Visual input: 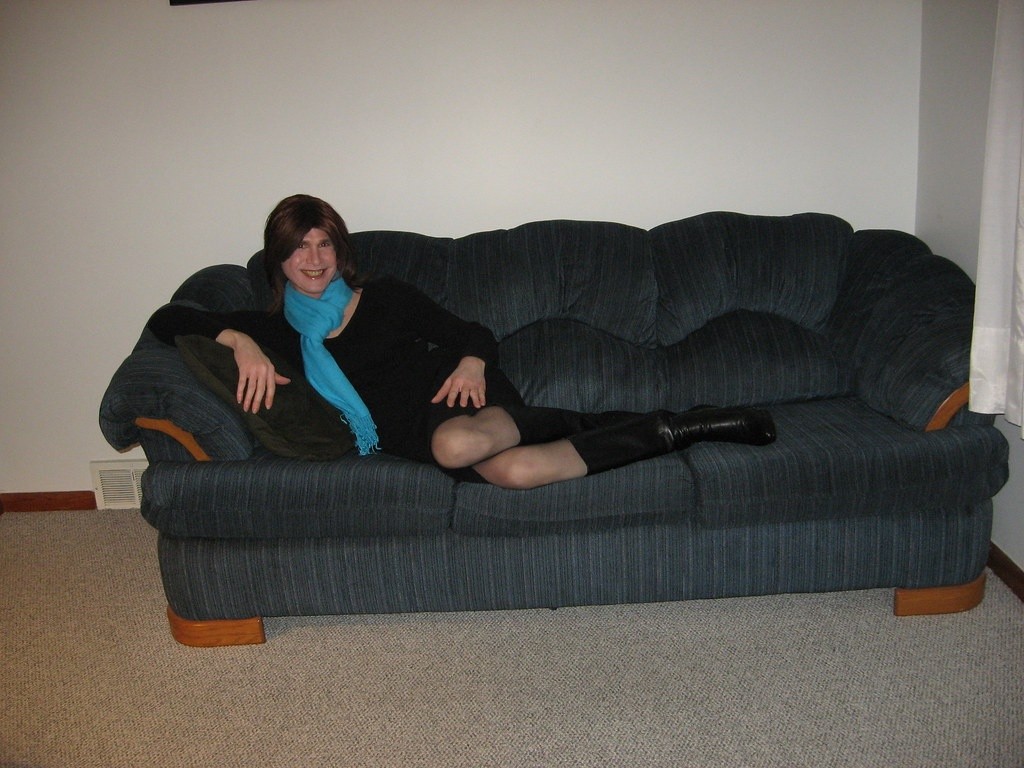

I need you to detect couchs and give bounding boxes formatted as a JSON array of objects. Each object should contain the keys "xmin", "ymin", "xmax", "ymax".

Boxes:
[{"xmin": 98, "ymin": 210, "xmax": 1010, "ymax": 648}]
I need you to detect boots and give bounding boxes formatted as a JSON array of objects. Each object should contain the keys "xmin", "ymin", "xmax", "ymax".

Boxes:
[
  {"xmin": 513, "ymin": 405, "xmax": 721, "ymax": 447},
  {"xmin": 568, "ymin": 404, "xmax": 777, "ymax": 478}
]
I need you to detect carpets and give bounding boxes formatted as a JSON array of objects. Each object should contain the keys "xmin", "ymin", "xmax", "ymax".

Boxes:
[{"xmin": 0, "ymin": 508, "xmax": 1022, "ymax": 767}]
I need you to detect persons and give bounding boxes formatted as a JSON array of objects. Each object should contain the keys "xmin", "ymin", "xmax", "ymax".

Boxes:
[{"xmin": 151, "ymin": 194, "xmax": 774, "ymax": 489}]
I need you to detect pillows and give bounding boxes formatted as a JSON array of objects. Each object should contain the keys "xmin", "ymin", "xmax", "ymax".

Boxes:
[{"xmin": 174, "ymin": 333, "xmax": 357, "ymax": 462}]
